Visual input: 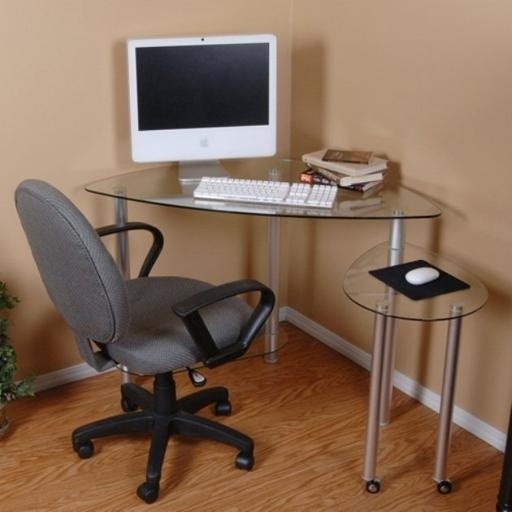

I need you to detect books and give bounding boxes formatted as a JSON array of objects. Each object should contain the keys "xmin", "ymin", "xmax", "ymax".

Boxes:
[
  {"xmin": 302, "ymin": 149, "xmax": 389, "ymax": 177},
  {"xmin": 338, "ymin": 183, "xmax": 388, "ymax": 217},
  {"xmin": 321, "ymin": 149, "xmax": 375, "ymax": 166},
  {"xmin": 306, "ymin": 162, "xmax": 383, "ymax": 188},
  {"xmin": 300, "ymin": 168, "xmax": 382, "ymax": 193}
]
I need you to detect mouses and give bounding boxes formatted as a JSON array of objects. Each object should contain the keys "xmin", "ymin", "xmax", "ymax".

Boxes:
[{"xmin": 405, "ymin": 266, "xmax": 440, "ymax": 285}]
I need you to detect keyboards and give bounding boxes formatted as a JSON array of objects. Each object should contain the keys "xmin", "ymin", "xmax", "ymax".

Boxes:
[{"xmin": 193, "ymin": 176, "xmax": 340, "ymax": 208}]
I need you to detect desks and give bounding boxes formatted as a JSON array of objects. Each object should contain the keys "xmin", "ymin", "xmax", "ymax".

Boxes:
[{"xmin": 84, "ymin": 156, "xmax": 490, "ymax": 494}]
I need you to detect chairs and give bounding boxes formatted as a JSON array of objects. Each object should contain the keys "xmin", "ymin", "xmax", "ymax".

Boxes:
[{"xmin": 14, "ymin": 178, "xmax": 276, "ymax": 502}]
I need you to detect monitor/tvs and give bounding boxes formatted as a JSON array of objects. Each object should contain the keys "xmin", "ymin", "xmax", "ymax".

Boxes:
[{"xmin": 128, "ymin": 35, "xmax": 277, "ymax": 181}]
